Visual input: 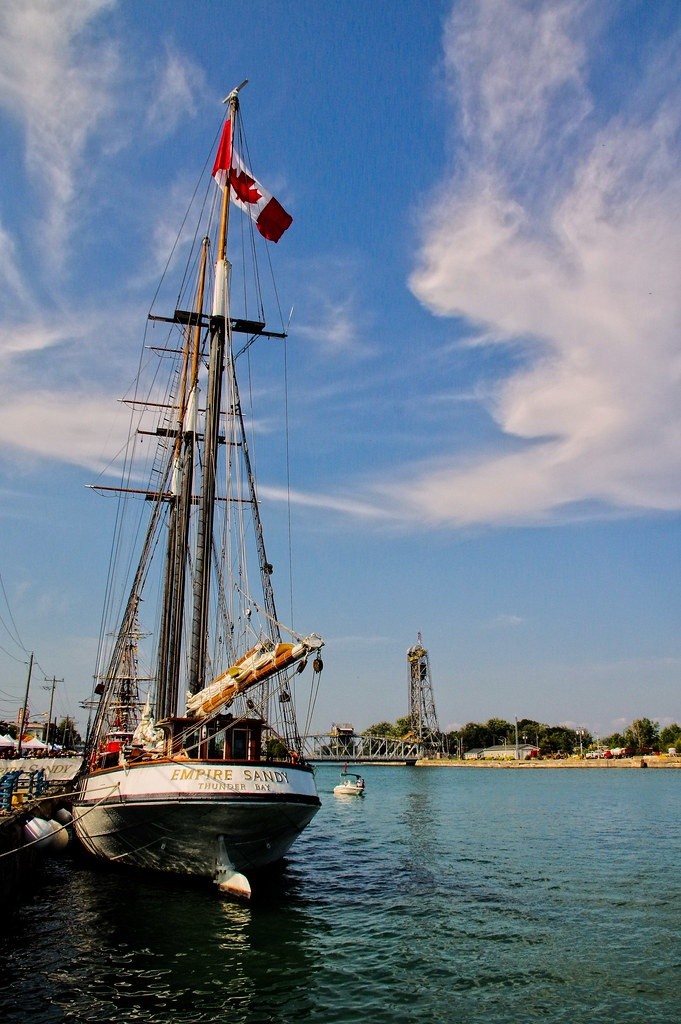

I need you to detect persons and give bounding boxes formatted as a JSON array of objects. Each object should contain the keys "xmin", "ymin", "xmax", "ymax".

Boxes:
[{"xmin": 0, "ymin": 749, "xmax": 81, "ymax": 760}]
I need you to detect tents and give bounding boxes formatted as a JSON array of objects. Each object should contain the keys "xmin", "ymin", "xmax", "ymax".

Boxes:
[{"xmin": 0, "ymin": 734, "xmax": 53, "ymax": 749}]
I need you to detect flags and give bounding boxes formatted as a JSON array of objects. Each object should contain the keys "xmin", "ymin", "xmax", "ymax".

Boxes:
[{"xmin": 210, "ymin": 113, "xmax": 293, "ymax": 243}]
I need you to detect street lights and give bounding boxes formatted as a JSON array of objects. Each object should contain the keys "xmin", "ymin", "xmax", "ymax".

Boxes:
[
  {"xmin": 18, "ymin": 712, "xmax": 48, "ymax": 756},
  {"xmin": 500, "ymin": 736, "xmax": 506, "ymax": 751},
  {"xmin": 576, "ymin": 727, "xmax": 584, "ymax": 759},
  {"xmin": 521, "ymin": 731, "xmax": 528, "ymax": 744}
]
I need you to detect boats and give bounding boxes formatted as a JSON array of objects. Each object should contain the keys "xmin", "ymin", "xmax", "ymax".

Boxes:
[{"xmin": 334, "ymin": 773, "xmax": 366, "ymax": 795}]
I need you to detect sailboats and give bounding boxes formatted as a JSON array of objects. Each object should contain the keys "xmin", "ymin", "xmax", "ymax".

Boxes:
[{"xmin": 71, "ymin": 78, "xmax": 321, "ymax": 900}]
